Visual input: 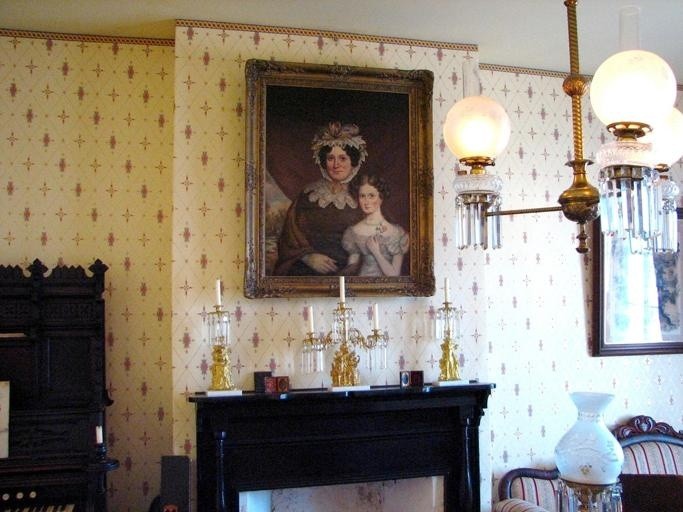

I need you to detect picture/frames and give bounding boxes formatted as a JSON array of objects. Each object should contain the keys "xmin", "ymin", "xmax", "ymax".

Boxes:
[
  {"xmin": 593, "ymin": 199, "xmax": 683, "ymax": 355},
  {"xmin": 242, "ymin": 56, "xmax": 439, "ymax": 298}
]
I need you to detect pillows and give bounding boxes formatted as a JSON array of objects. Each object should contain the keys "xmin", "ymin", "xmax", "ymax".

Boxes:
[{"xmin": 619, "ymin": 473, "xmax": 682, "ymax": 511}]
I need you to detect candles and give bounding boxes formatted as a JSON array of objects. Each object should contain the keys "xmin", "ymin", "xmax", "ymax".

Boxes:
[
  {"xmin": 338, "ymin": 276, "xmax": 345, "ymax": 302},
  {"xmin": 443, "ymin": 277, "xmax": 449, "ymax": 302},
  {"xmin": 372, "ymin": 302, "xmax": 379, "ymax": 329},
  {"xmin": 307, "ymin": 305, "xmax": 313, "ymax": 332},
  {"xmin": 214, "ymin": 279, "xmax": 221, "ymax": 305},
  {"xmin": 95, "ymin": 424, "xmax": 102, "ymax": 443}
]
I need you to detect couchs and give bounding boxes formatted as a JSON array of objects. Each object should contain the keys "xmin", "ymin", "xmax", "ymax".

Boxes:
[{"xmin": 492, "ymin": 415, "xmax": 682, "ymax": 511}]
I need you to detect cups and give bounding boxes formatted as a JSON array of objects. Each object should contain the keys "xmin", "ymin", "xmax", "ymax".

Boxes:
[{"xmin": 255, "ymin": 371, "xmax": 271, "ymax": 392}]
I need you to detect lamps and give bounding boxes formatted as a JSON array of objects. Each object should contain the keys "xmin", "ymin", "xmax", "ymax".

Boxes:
[{"xmin": 437, "ymin": 0, "xmax": 683, "ymax": 254}]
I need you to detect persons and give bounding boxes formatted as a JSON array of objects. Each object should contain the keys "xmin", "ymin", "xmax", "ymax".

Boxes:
[
  {"xmin": 273, "ymin": 121, "xmax": 369, "ymax": 275},
  {"xmin": 340, "ymin": 174, "xmax": 410, "ymax": 276}
]
